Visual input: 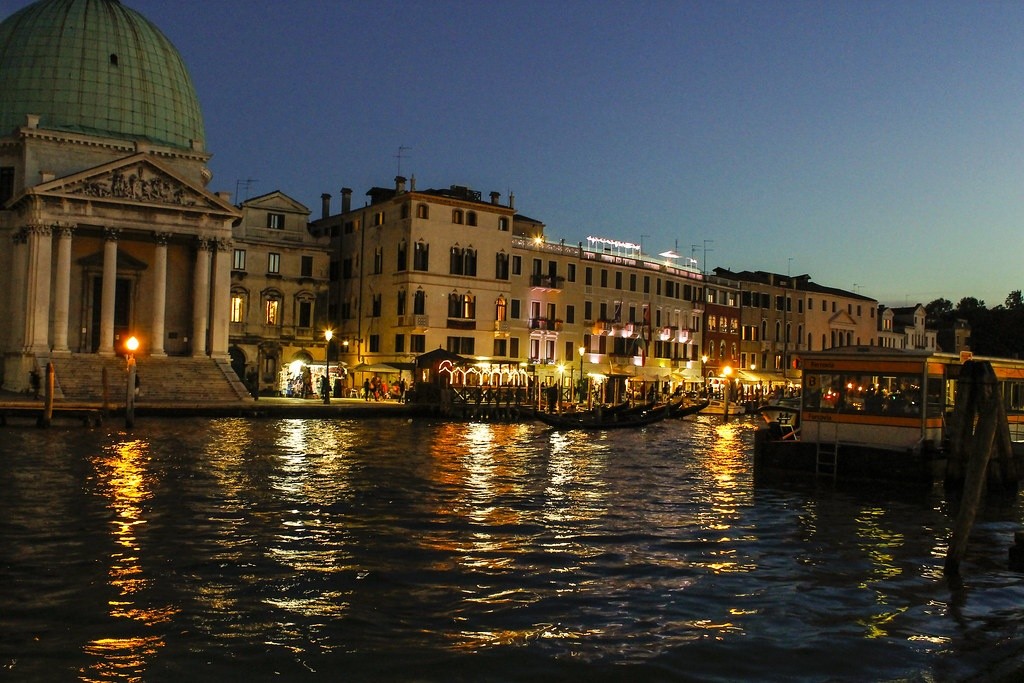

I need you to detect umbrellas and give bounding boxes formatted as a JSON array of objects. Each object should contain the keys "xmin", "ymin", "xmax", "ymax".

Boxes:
[
  {"xmin": 631, "ymin": 373, "xmax": 704, "ymax": 392},
  {"xmin": 346, "ymin": 362, "xmax": 400, "ymax": 382}
]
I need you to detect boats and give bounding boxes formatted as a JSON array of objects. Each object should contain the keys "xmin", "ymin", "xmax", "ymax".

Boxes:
[
  {"xmin": 754, "ymin": 345, "xmax": 1024, "ymax": 483},
  {"xmin": 515, "ymin": 397, "xmax": 709, "ymax": 430}
]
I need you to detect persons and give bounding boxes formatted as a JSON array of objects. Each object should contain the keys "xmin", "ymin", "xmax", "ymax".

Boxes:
[
  {"xmin": 320, "ymin": 376, "xmax": 332, "ymax": 399},
  {"xmin": 864, "ymin": 380, "xmax": 921, "ymax": 415},
  {"xmin": 638, "ymin": 383, "xmax": 713, "ymax": 403},
  {"xmin": 78, "ymin": 173, "xmax": 196, "ymax": 206},
  {"xmin": 126, "ymin": 370, "xmax": 140, "ymax": 402},
  {"xmin": 363, "ymin": 376, "xmax": 407, "ymax": 404},
  {"xmin": 546, "ymin": 383, "xmax": 558, "ymax": 416}
]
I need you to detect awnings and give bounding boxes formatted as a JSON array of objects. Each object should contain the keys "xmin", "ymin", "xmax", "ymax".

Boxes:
[{"xmin": 740, "ymin": 371, "xmax": 791, "ymax": 385}]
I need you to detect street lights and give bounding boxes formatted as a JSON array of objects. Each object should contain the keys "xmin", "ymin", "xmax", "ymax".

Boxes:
[
  {"xmin": 579, "ymin": 346, "xmax": 585, "ymax": 404},
  {"xmin": 325, "ymin": 328, "xmax": 333, "ymax": 404}
]
